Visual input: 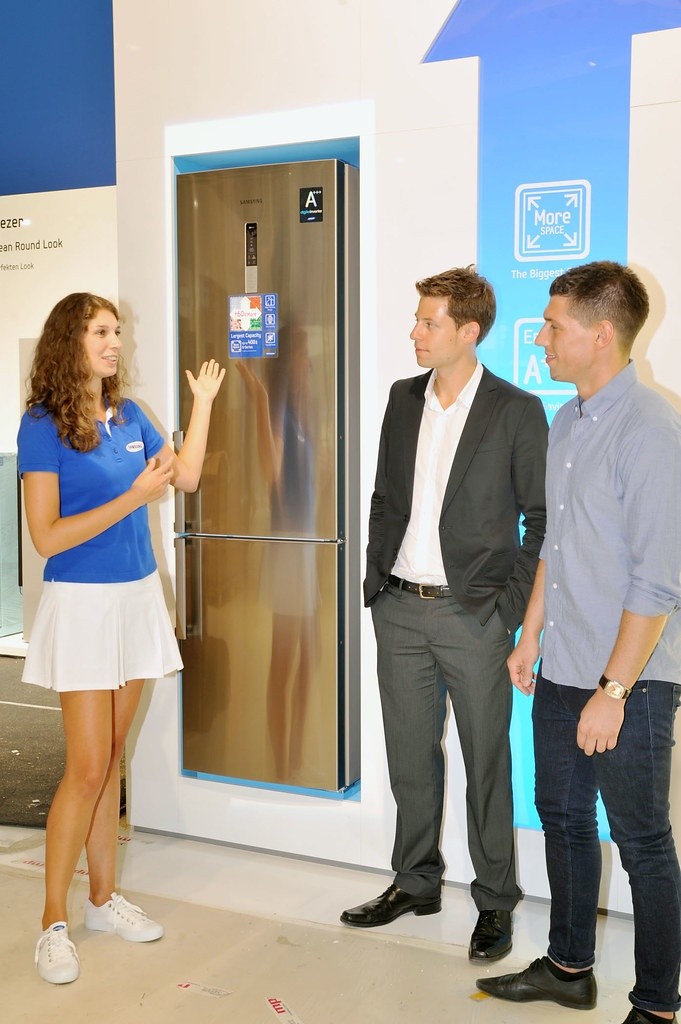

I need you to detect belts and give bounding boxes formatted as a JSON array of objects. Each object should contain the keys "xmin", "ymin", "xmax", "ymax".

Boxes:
[{"xmin": 388, "ymin": 574, "xmax": 454, "ymax": 597}]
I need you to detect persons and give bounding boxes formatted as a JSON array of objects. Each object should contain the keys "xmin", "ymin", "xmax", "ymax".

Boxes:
[
  {"xmin": 340, "ymin": 267, "xmax": 550, "ymax": 966},
  {"xmin": 235, "ymin": 325, "xmax": 319, "ymax": 786},
  {"xmin": 478, "ymin": 262, "xmax": 681, "ymax": 1024},
  {"xmin": 16, "ymin": 292, "xmax": 226, "ymax": 984}
]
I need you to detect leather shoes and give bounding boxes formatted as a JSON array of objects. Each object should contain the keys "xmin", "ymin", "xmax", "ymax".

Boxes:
[
  {"xmin": 340, "ymin": 883, "xmax": 444, "ymax": 928},
  {"xmin": 467, "ymin": 909, "xmax": 517, "ymax": 963},
  {"xmin": 476, "ymin": 956, "xmax": 599, "ymax": 1009},
  {"xmin": 621, "ymin": 1010, "xmax": 680, "ymax": 1024}
]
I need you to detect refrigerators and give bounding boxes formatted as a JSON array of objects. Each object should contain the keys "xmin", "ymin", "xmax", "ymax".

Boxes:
[{"xmin": 173, "ymin": 157, "xmax": 360, "ymax": 793}]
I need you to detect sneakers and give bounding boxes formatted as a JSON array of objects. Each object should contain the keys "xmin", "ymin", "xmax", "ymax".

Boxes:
[
  {"xmin": 34, "ymin": 921, "xmax": 80, "ymax": 985},
  {"xmin": 86, "ymin": 894, "xmax": 164, "ymax": 942}
]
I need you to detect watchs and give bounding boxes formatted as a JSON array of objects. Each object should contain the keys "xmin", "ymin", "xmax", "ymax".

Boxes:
[{"xmin": 599, "ymin": 674, "xmax": 630, "ymax": 701}]
